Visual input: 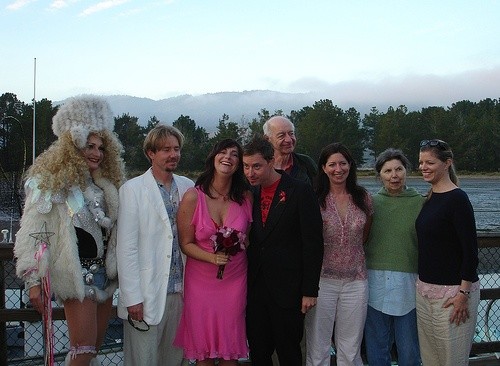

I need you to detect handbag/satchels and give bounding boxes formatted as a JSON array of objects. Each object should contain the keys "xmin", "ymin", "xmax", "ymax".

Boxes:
[{"xmin": 80, "ymin": 258, "xmax": 109, "ymax": 290}]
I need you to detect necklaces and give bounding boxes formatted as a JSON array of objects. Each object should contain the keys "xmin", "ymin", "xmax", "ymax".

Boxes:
[
  {"xmin": 158, "ymin": 176, "xmax": 178, "ymax": 200},
  {"xmin": 211, "ymin": 185, "xmax": 230, "ymax": 202}
]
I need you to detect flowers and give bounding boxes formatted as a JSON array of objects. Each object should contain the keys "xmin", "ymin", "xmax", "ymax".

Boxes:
[
  {"xmin": 211, "ymin": 226, "xmax": 249, "ymax": 279},
  {"xmin": 274, "ymin": 189, "xmax": 288, "ymax": 210}
]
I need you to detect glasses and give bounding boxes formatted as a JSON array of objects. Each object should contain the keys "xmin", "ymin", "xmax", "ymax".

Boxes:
[
  {"xmin": 419, "ymin": 139, "xmax": 447, "ymax": 151},
  {"xmin": 127, "ymin": 313, "xmax": 150, "ymax": 332}
]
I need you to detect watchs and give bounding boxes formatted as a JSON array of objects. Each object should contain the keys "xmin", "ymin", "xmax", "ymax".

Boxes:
[{"xmin": 460, "ymin": 290, "xmax": 470, "ymax": 296}]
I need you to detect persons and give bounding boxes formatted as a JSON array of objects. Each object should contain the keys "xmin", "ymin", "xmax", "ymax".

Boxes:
[
  {"xmin": 117, "ymin": 126, "xmax": 195, "ymax": 366},
  {"xmin": 364, "ymin": 149, "xmax": 427, "ymax": 366},
  {"xmin": 263, "ymin": 116, "xmax": 318, "ymax": 188},
  {"xmin": 305, "ymin": 145, "xmax": 374, "ymax": 366},
  {"xmin": 243, "ymin": 139, "xmax": 323, "ymax": 366},
  {"xmin": 176, "ymin": 138, "xmax": 253, "ymax": 366},
  {"xmin": 415, "ymin": 141, "xmax": 480, "ymax": 366},
  {"xmin": 15, "ymin": 132, "xmax": 126, "ymax": 366}
]
